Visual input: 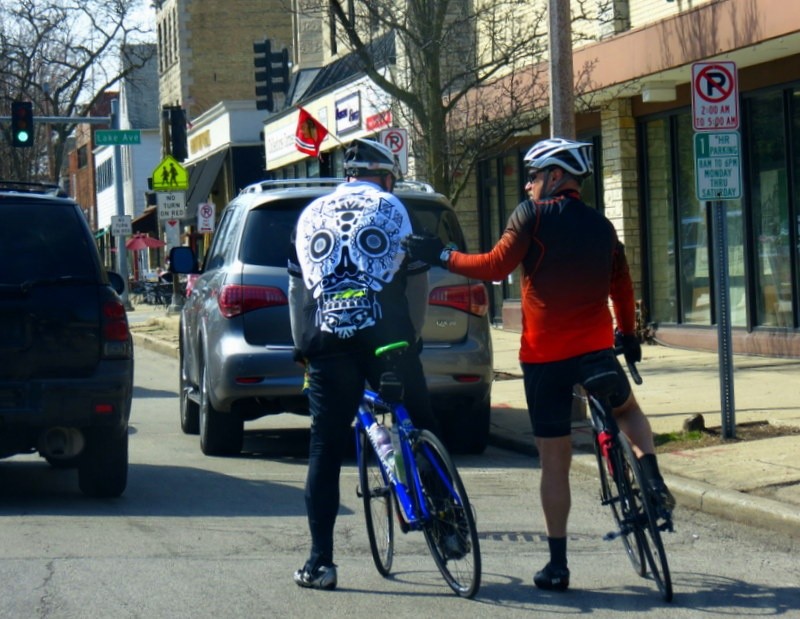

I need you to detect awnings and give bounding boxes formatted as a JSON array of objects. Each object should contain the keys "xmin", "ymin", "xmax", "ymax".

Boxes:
[{"xmin": 180, "ymin": 148, "xmax": 227, "ymax": 226}]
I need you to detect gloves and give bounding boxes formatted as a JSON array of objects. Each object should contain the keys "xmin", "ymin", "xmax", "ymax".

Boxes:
[
  {"xmin": 401, "ymin": 233, "xmax": 452, "ymax": 270},
  {"xmin": 615, "ymin": 327, "xmax": 635, "ymax": 348}
]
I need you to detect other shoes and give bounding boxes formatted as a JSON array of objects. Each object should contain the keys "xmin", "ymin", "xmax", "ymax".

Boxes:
[
  {"xmin": 646, "ymin": 478, "xmax": 675, "ymax": 517},
  {"xmin": 534, "ymin": 564, "xmax": 570, "ymax": 590},
  {"xmin": 438, "ymin": 527, "xmax": 466, "ymax": 559}
]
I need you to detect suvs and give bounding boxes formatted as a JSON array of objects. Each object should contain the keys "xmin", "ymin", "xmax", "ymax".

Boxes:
[
  {"xmin": 1, "ymin": 179, "xmax": 135, "ymax": 498},
  {"xmin": 165, "ymin": 176, "xmax": 496, "ymax": 459}
]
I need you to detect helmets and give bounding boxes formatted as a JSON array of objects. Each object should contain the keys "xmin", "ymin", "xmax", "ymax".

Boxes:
[
  {"xmin": 343, "ymin": 136, "xmax": 402, "ymax": 180},
  {"xmin": 523, "ymin": 138, "xmax": 594, "ymax": 178}
]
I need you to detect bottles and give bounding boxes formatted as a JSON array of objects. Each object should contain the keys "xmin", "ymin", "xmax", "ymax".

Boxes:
[
  {"xmin": 393, "ymin": 450, "xmax": 406, "ymax": 484},
  {"xmin": 377, "ymin": 424, "xmax": 395, "ymax": 466}
]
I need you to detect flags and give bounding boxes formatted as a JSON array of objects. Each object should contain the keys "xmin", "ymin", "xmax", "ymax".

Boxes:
[{"xmin": 294, "ymin": 109, "xmax": 327, "ymax": 156}]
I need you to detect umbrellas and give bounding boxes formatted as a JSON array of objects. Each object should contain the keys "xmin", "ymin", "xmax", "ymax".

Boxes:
[{"xmin": 113, "ymin": 234, "xmax": 167, "ymax": 267}]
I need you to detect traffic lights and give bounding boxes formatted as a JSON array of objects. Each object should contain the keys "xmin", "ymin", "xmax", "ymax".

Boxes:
[
  {"xmin": 270, "ymin": 47, "xmax": 291, "ymax": 95},
  {"xmin": 11, "ymin": 100, "xmax": 34, "ymax": 148},
  {"xmin": 169, "ymin": 105, "xmax": 189, "ymax": 162},
  {"xmin": 252, "ymin": 38, "xmax": 275, "ymax": 113}
]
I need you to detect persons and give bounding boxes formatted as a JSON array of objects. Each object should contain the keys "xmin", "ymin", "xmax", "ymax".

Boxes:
[
  {"xmin": 288, "ymin": 139, "xmax": 471, "ymax": 589},
  {"xmin": 401, "ymin": 139, "xmax": 674, "ymax": 589}
]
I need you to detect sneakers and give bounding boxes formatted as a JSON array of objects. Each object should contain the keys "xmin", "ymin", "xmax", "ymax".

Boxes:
[{"xmin": 294, "ymin": 559, "xmax": 338, "ymax": 591}]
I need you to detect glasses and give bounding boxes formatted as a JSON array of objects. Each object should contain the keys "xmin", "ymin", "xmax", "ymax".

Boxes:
[{"xmin": 526, "ymin": 172, "xmax": 538, "ymax": 183}]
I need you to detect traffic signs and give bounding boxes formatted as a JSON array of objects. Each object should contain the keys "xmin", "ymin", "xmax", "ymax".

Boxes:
[{"xmin": 196, "ymin": 202, "xmax": 215, "ymax": 234}]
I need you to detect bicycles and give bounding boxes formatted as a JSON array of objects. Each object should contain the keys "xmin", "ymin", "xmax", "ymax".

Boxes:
[
  {"xmin": 574, "ymin": 318, "xmax": 678, "ymax": 605},
  {"xmin": 293, "ymin": 338, "xmax": 483, "ymax": 600}
]
